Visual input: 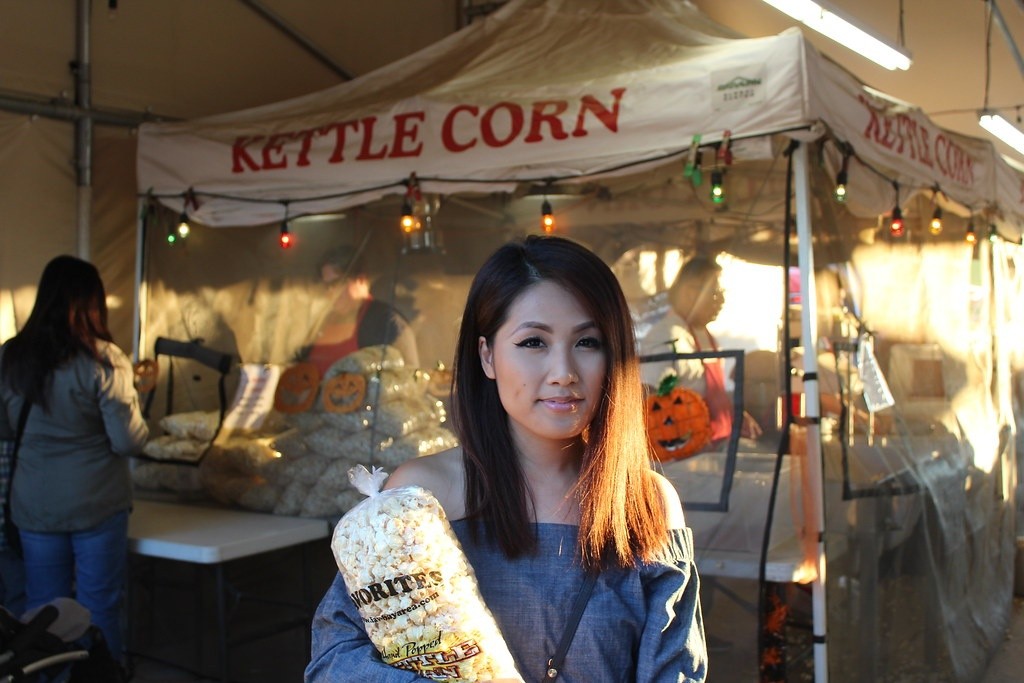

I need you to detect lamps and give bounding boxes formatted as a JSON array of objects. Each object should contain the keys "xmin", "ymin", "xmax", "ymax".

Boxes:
[
  {"xmin": 760, "ymin": 0, "xmax": 914, "ymax": 70},
  {"xmin": 977, "ymin": 1, "xmax": 1023, "ymax": 155}
]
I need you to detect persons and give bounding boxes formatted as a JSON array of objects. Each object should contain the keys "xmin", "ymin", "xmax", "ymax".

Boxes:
[
  {"xmin": 305, "ymin": 234, "xmax": 709, "ymax": 683},
  {"xmin": 640, "ymin": 256, "xmax": 762, "ymax": 451},
  {"xmin": 261, "ymin": 244, "xmax": 419, "ymax": 436},
  {"xmin": 0, "ymin": 254, "xmax": 148, "ymax": 672}
]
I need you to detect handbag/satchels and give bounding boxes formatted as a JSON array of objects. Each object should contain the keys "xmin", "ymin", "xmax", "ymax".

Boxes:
[{"xmin": 3, "ymin": 503, "xmax": 25, "ymax": 561}]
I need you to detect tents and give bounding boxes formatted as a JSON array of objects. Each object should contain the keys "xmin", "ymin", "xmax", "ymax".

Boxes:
[{"xmin": 131, "ymin": 0, "xmax": 1024, "ymax": 683}]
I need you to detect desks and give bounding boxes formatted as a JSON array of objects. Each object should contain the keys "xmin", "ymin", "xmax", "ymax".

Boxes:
[
  {"xmin": 124, "ymin": 499, "xmax": 329, "ymax": 683},
  {"xmin": 655, "ymin": 435, "xmax": 968, "ymax": 683}
]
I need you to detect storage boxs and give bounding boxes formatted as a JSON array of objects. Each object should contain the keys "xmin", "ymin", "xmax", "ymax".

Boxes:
[{"xmin": 664, "ymin": 452, "xmax": 807, "ymax": 551}]
